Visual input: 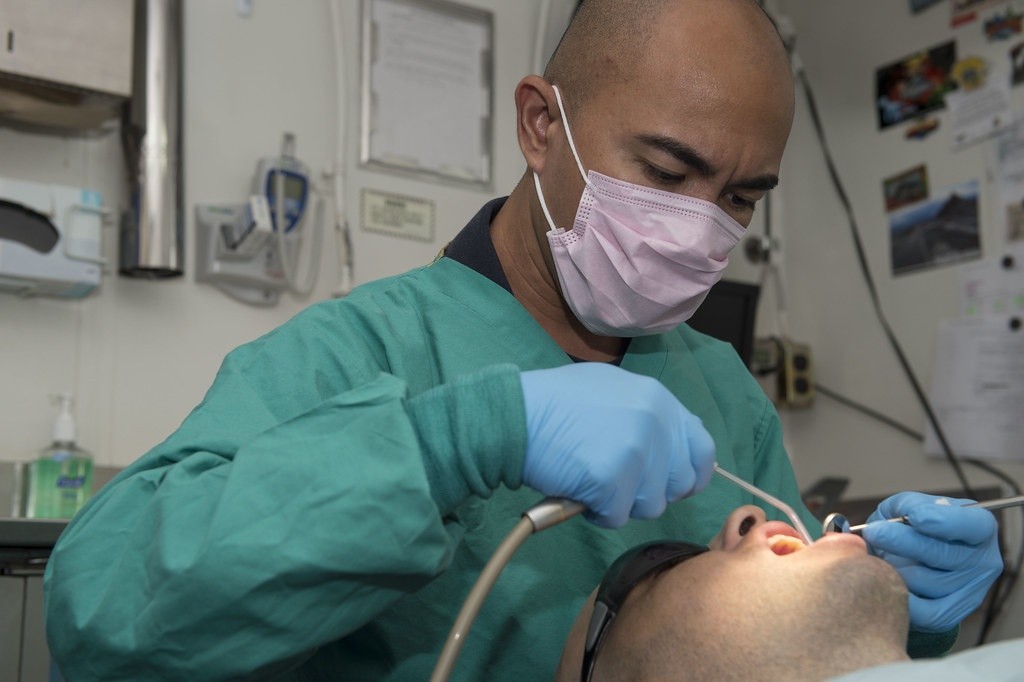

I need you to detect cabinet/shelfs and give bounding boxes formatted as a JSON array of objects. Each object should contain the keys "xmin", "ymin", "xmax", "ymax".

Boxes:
[{"xmin": 0, "ymin": 0, "xmax": 135, "ymax": 131}]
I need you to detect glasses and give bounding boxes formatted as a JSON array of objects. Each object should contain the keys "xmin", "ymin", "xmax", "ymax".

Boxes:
[{"xmin": 580, "ymin": 539, "xmax": 710, "ymax": 682}]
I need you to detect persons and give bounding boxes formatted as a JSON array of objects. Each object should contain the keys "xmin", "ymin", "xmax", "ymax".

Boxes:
[{"xmin": 41, "ymin": 1, "xmax": 1024, "ymax": 682}]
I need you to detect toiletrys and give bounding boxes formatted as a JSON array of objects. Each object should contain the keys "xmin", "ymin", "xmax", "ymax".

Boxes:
[{"xmin": 28, "ymin": 393, "xmax": 94, "ymax": 522}]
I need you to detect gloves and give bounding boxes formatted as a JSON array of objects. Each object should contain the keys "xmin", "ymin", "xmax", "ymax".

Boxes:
[
  {"xmin": 519, "ymin": 363, "xmax": 718, "ymax": 529},
  {"xmin": 862, "ymin": 492, "xmax": 1004, "ymax": 635}
]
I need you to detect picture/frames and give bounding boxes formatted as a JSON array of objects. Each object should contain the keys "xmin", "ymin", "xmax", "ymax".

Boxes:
[{"xmin": 356, "ymin": 2, "xmax": 496, "ymax": 194}]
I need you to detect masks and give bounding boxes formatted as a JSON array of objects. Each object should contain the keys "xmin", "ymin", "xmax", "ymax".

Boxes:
[{"xmin": 537, "ymin": 84, "xmax": 747, "ymax": 338}]
